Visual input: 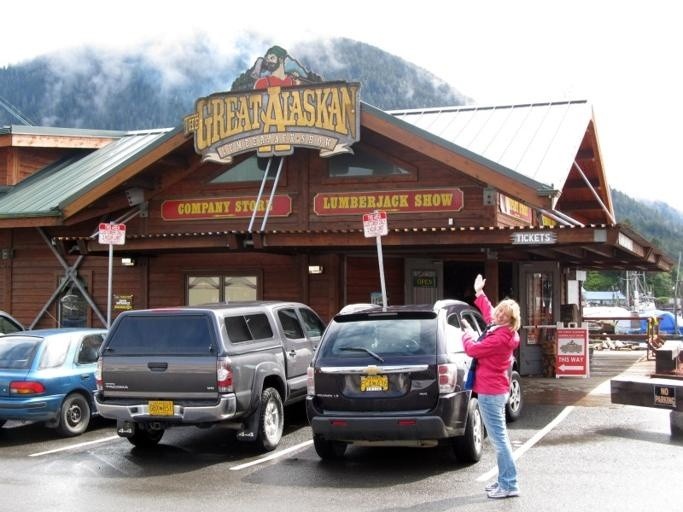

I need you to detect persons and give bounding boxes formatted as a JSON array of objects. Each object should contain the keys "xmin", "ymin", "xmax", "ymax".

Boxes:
[
  {"xmin": 255, "ymin": 46, "xmax": 297, "ymax": 91},
  {"xmin": 462, "ymin": 273, "xmax": 522, "ymax": 499}
]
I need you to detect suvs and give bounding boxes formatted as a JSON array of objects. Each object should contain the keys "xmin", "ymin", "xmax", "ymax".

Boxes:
[{"xmin": 305, "ymin": 297, "xmax": 526, "ymax": 464}]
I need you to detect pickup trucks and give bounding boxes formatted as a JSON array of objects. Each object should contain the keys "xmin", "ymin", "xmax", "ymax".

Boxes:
[{"xmin": 90, "ymin": 300, "xmax": 327, "ymax": 454}]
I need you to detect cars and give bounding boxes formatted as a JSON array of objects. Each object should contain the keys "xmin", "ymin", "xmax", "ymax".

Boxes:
[
  {"xmin": 1, "ymin": 327, "xmax": 111, "ymax": 438},
  {"xmin": 1, "ymin": 309, "xmax": 27, "ymax": 337},
  {"xmin": 581, "ymin": 304, "xmax": 628, "ymax": 334},
  {"xmin": 615, "ymin": 309, "xmax": 683, "ymax": 337}
]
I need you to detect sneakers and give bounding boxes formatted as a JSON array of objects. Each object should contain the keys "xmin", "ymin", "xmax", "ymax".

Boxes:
[{"xmin": 486, "ymin": 483, "xmax": 517, "ymax": 498}]
